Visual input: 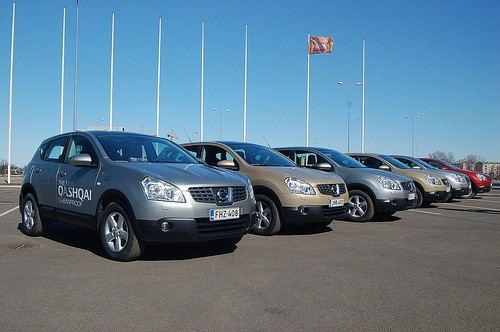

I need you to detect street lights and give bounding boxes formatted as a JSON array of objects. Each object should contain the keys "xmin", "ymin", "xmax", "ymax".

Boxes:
[
  {"xmin": 213, "ymin": 109, "xmax": 230, "ymax": 141},
  {"xmin": 338, "ymin": 82, "xmax": 362, "ymax": 154},
  {"xmin": 406, "ymin": 116, "xmax": 420, "ymax": 157}
]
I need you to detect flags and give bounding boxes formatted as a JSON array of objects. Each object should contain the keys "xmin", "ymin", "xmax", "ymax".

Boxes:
[{"xmin": 310, "ymin": 36, "xmax": 334, "ymax": 54}]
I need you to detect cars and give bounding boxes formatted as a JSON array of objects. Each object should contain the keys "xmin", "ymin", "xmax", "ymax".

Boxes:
[
  {"xmin": 403, "ymin": 158, "xmax": 493, "ymax": 199},
  {"xmin": 365, "ymin": 154, "xmax": 472, "ymax": 202},
  {"xmin": 234, "ymin": 146, "xmax": 417, "ymax": 222},
  {"xmin": 158, "ymin": 142, "xmax": 350, "ymax": 236},
  {"xmin": 18, "ymin": 130, "xmax": 257, "ymax": 262},
  {"xmin": 331, "ymin": 153, "xmax": 452, "ymax": 208}
]
